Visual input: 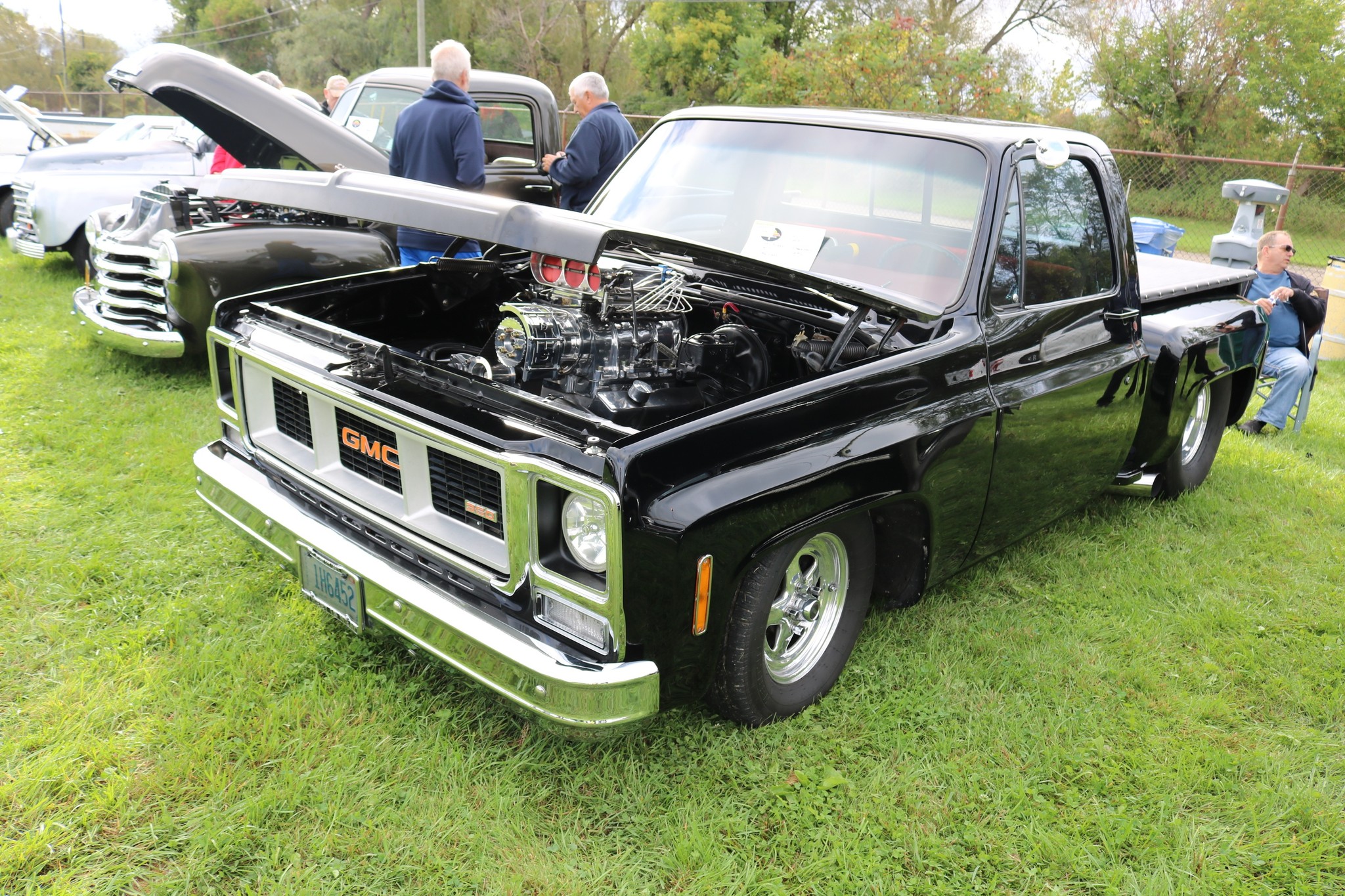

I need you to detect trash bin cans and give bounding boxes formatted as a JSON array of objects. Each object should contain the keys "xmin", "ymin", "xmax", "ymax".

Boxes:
[
  {"xmin": 1211, "ymin": 178, "xmax": 1291, "ymax": 274},
  {"xmin": 1129, "ymin": 215, "xmax": 1186, "ymax": 258}
]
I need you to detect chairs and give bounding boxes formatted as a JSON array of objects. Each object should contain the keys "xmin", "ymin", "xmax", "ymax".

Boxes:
[{"xmin": 1256, "ymin": 286, "xmax": 1330, "ymax": 433}]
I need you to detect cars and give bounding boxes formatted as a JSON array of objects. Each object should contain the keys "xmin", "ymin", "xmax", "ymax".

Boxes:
[
  {"xmin": 69, "ymin": 42, "xmax": 564, "ymax": 361},
  {"xmin": 185, "ymin": 102, "xmax": 1276, "ymax": 747},
  {"xmin": 0, "ymin": 82, "xmax": 218, "ymax": 279}
]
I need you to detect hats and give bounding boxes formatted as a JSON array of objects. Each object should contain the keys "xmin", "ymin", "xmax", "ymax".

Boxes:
[{"xmin": 326, "ymin": 74, "xmax": 349, "ymax": 97}]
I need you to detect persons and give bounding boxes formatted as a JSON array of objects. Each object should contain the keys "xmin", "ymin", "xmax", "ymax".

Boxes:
[
  {"xmin": 320, "ymin": 75, "xmax": 350, "ymax": 116},
  {"xmin": 541, "ymin": 71, "xmax": 639, "ymax": 213},
  {"xmin": 389, "ymin": 37, "xmax": 486, "ymax": 266},
  {"xmin": 211, "ymin": 70, "xmax": 283, "ymax": 205},
  {"xmin": 1238, "ymin": 230, "xmax": 1325, "ymax": 436}
]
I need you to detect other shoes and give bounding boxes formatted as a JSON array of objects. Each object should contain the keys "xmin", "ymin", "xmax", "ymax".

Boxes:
[{"xmin": 1238, "ymin": 419, "xmax": 1266, "ymax": 435}]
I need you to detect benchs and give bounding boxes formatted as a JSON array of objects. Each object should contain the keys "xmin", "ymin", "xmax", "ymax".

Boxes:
[{"xmin": 750, "ymin": 222, "xmax": 1084, "ymax": 303}]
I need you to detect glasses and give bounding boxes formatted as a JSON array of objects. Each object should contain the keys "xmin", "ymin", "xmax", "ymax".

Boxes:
[{"xmin": 1261, "ymin": 245, "xmax": 1296, "ymax": 256}]
